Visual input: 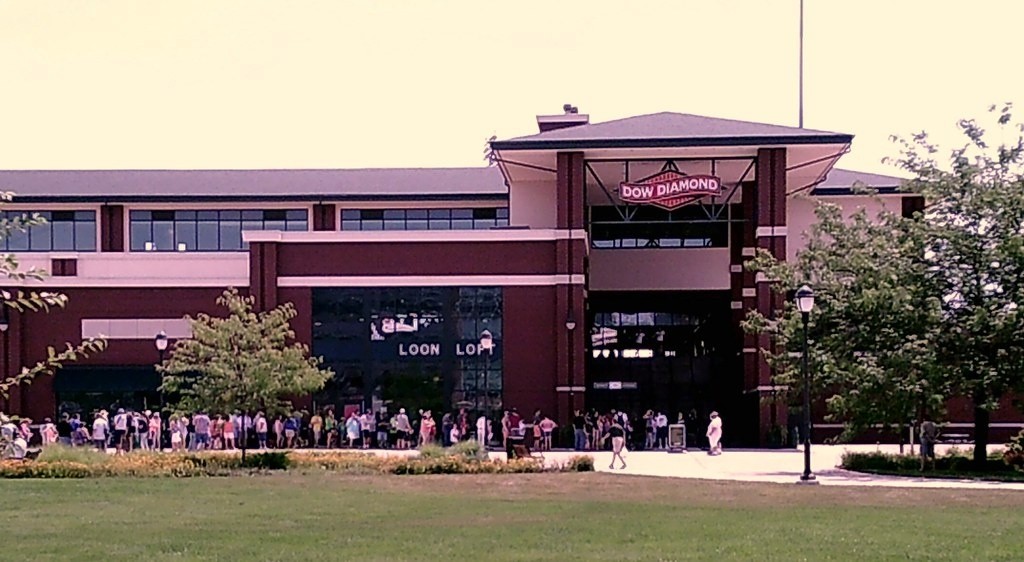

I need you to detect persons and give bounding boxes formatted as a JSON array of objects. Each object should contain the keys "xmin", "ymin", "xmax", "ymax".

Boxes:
[
  {"xmin": 600, "ymin": 417, "xmax": 628, "ymax": 470},
  {"xmin": 644, "ymin": 406, "xmax": 694, "ymax": 448},
  {"xmin": 571, "ymin": 406, "xmax": 632, "ymax": 452},
  {"xmin": 442, "ymin": 408, "xmax": 558, "ymax": 458},
  {"xmin": 705, "ymin": 411, "xmax": 724, "ymax": 455},
  {"xmin": 0, "ymin": 407, "xmax": 437, "ymax": 461},
  {"xmin": 919, "ymin": 422, "xmax": 936, "ymax": 472}
]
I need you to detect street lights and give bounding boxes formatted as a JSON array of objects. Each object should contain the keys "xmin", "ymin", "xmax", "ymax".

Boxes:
[
  {"xmin": 481, "ymin": 330, "xmax": 492, "ymax": 447},
  {"xmin": 154, "ymin": 331, "xmax": 169, "ymax": 451},
  {"xmin": 795, "ymin": 283, "xmax": 817, "ymax": 480}
]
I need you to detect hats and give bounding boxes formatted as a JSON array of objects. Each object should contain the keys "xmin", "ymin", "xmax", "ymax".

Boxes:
[
  {"xmin": 20, "ymin": 418, "xmax": 34, "ymax": 424},
  {"xmin": 9, "ymin": 415, "xmax": 20, "ymax": 422}
]
